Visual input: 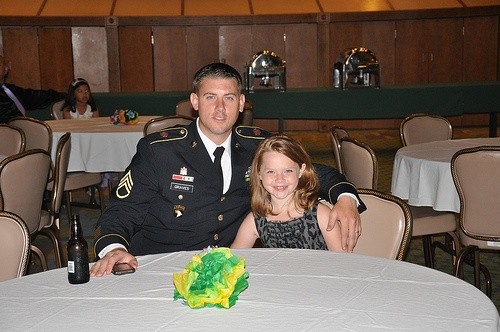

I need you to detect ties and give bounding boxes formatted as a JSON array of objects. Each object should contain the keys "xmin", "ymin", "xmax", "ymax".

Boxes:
[
  {"xmin": 213, "ymin": 146, "xmax": 225, "ymax": 197},
  {"xmin": 2, "ymin": 84, "xmax": 25, "ymax": 116}
]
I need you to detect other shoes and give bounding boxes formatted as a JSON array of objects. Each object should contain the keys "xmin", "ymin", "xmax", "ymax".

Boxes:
[{"xmin": 86, "ymin": 186, "xmax": 98, "ymax": 196}]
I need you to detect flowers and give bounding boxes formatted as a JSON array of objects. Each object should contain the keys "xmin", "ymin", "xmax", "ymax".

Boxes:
[{"xmin": 110, "ymin": 109, "xmax": 140, "ymax": 125}]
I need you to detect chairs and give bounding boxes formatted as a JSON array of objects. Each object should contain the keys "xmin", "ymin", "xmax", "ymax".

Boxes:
[{"xmin": 0, "ymin": 115, "xmax": 500, "ymax": 300}]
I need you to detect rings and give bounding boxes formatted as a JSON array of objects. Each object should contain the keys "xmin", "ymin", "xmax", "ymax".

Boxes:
[{"xmin": 355, "ymin": 231, "xmax": 359, "ymax": 233}]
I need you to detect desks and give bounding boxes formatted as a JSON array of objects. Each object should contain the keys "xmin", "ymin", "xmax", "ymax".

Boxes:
[
  {"xmin": 44, "ymin": 116, "xmax": 164, "ymax": 210},
  {"xmin": 0, "ymin": 248, "xmax": 500, "ymax": 332},
  {"xmin": 391, "ymin": 137, "xmax": 500, "ymax": 271}
]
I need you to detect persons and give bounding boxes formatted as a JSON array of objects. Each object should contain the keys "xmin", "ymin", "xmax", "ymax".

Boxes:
[
  {"xmin": 89, "ymin": 63, "xmax": 367, "ymax": 277},
  {"xmin": 61, "ymin": 79, "xmax": 98, "ymax": 120},
  {"xmin": 231, "ymin": 135, "xmax": 349, "ymax": 251},
  {"xmin": 0, "ymin": 55, "xmax": 66, "ymax": 124}
]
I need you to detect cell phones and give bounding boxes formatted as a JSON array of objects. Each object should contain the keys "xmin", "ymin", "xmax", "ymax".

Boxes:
[{"xmin": 111, "ymin": 262, "xmax": 135, "ymax": 275}]
